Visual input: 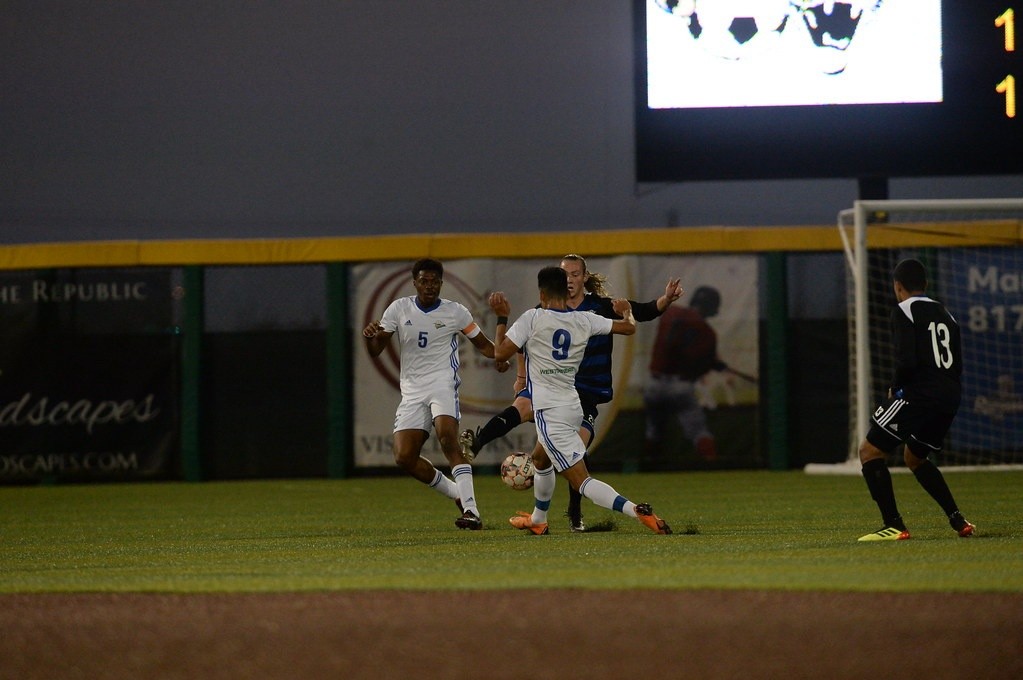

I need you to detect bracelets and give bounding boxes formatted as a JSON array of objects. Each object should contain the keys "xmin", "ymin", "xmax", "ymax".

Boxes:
[
  {"xmin": 517, "ymin": 375, "xmax": 526, "ymax": 378},
  {"xmin": 364, "ymin": 332, "xmax": 375, "ymax": 339},
  {"xmin": 497, "ymin": 316, "xmax": 508, "ymax": 326}
]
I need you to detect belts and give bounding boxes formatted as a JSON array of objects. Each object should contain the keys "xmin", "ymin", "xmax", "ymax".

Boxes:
[{"xmin": 652, "ymin": 372, "xmax": 697, "ymax": 380}]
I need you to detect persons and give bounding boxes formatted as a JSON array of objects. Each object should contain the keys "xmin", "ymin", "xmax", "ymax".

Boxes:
[
  {"xmin": 459, "ymin": 254, "xmax": 684, "ymax": 531},
  {"xmin": 488, "ymin": 266, "xmax": 673, "ymax": 535},
  {"xmin": 856, "ymin": 258, "xmax": 977, "ymax": 542},
  {"xmin": 639, "ymin": 286, "xmax": 727, "ymax": 470},
  {"xmin": 363, "ymin": 259, "xmax": 509, "ymax": 531}
]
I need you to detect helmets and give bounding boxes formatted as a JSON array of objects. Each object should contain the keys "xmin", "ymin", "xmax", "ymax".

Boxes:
[{"xmin": 689, "ymin": 285, "xmax": 720, "ymax": 316}]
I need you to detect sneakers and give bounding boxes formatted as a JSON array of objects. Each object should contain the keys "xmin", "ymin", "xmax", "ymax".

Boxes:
[
  {"xmin": 950, "ymin": 514, "xmax": 977, "ymax": 537},
  {"xmin": 455, "ymin": 510, "xmax": 482, "ymax": 530},
  {"xmin": 563, "ymin": 509, "xmax": 586, "ymax": 532},
  {"xmin": 858, "ymin": 517, "xmax": 911, "ymax": 541},
  {"xmin": 455, "ymin": 498, "xmax": 464, "ymax": 515},
  {"xmin": 633, "ymin": 503, "xmax": 673, "ymax": 534},
  {"xmin": 459, "ymin": 425, "xmax": 481, "ymax": 461},
  {"xmin": 509, "ymin": 511, "xmax": 548, "ymax": 535}
]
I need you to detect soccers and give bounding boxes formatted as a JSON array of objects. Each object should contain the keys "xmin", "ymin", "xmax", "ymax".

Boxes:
[{"xmin": 500, "ymin": 451, "xmax": 535, "ymax": 491}]
